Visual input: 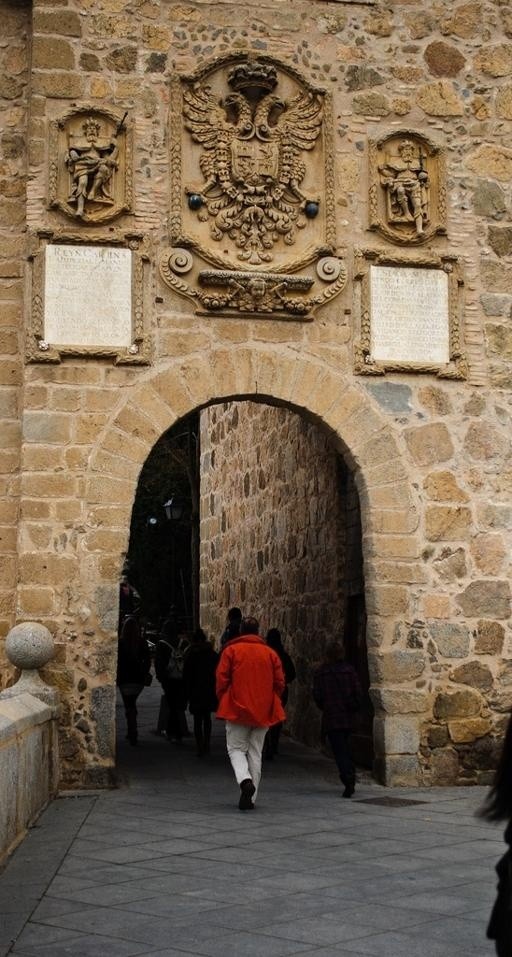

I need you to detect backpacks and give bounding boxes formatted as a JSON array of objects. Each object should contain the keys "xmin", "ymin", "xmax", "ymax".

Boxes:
[{"xmin": 159, "ymin": 637, "xmax": 191, "ymax": 681}]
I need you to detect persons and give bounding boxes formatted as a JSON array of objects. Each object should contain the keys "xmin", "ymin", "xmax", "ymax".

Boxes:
[
  {"xmin": 118, "ymin": 598, "xmax": 220, "ymax": 759},
  {"xmin": 311, "ymin": 641, "xmax": 375, "ymax": 799},
  {"xmin": 470, "ymin": 716, "xmax": 512, "ymax": 956},
  {"xmin": 219, "ymin": 608, "xmax": 245, "ymax": 649},
  {"xmin": 262, "ymin": 629, "xmax": 296, "ymax": 768},
  {"xmin": 214, "ymin": 616, "xmax": 288, "ymax": 809}
]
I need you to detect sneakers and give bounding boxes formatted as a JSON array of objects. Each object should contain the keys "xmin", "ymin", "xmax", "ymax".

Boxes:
[
  {"xmin": 237, "ymin": 778, "xmax": 258, "ymax": 810},
  {"xmin": 342, "ymin": 783, "xmax": 357, "ymax": 799}
]
